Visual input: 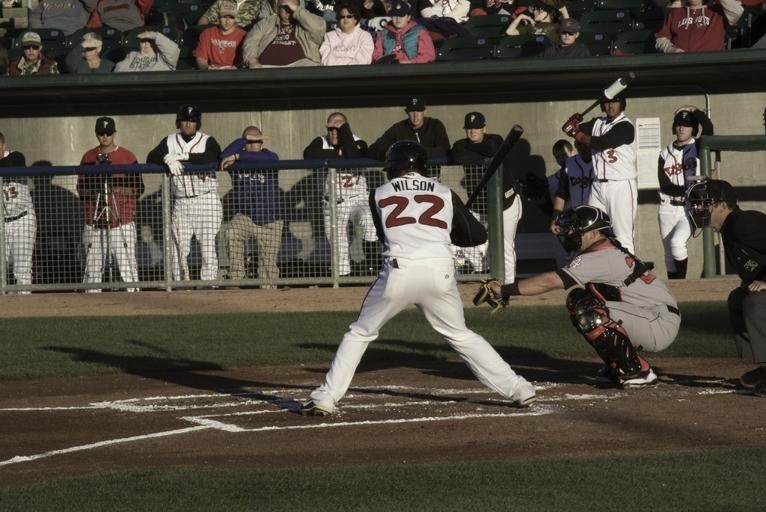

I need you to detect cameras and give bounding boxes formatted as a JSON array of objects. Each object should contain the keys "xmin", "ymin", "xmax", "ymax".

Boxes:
[{"xmin": 97, "ymin": 153, "xmax": 111, "ymax": 161}]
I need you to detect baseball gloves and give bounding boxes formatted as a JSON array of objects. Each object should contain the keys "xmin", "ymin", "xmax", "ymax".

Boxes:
[{"xmin": 473, "ymin": 278, "xmax": 509, "ymax": 314}]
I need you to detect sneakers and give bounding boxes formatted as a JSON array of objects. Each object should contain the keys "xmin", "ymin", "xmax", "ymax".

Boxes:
[
  {"xmin": 740, "ymin": 366, "xmax": 766, "ymax": 388},
  {"xmin": 296, "ymin": 398, "xmax": 329, "ymax": 416},
  {"xmin": 522, "ymin": 379, "xmax": 537, "ymax": 408},
  {"xmin": 583, "ymin": 366, "xmax": 615, "ymax": 383},
  {"xmin": 623, "ymin": 367, "xmax": 659, "ymax": 389}
]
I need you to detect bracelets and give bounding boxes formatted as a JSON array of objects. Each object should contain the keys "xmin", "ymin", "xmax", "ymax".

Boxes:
[
  {"xmin": 497, "ymin": 282, "xmax": 521, "ymax": 299},
  {"xmin": 575, "ymin": 131, "xmax": 585, "ymax": 141}
]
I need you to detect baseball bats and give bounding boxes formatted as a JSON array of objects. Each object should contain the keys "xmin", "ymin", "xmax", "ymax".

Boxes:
[
  {"xmin": 466, "ymin": 124, "xmax": 523, "ymax": 209},
  {"xmin": 579, "ymin": 72, "xmax": 635, "ymax": 121}
]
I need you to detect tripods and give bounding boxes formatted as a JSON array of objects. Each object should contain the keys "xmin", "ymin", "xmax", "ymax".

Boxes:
[{"xmin": 80, "ymin": 181, "xmax": 138, "ymax": 293}]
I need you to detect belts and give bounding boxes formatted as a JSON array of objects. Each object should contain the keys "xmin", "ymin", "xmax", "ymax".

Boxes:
[
  {"xmin": 668, "ymin": 305, "xmax": 680, "ymax": 314},
  {"xmin": 4, "ymin": 211, "xmax": 27, "ymax": 222},
  {"xmin": 671, "ymin": 200, "xmax": 685, "ymax": 205},
  {"xmin": 600, "ymin": 179, "xmax": 608, "ymax": 182}
]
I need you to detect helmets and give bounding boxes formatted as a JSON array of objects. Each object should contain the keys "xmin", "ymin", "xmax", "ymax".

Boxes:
[
  {"xmin": 382, "ymin": 141, "xmax": 428, "ymax": 180},
  {"xmin": 176, "ymin": 103, "xmax": 201, "ymax": 131},
  {"xmin": 600, "ymin": 87, "xmax": 627, "ymax": 112},
  {"xmin": 684, "ymin": 175, "xmax": 738, "ymax": 238},
  {"xmin": 555, "ymin": 204, "xmax": 616, "ymax": 263},
  {"xmin": 672, "ymin": 110, "xmax": 699, "ymax": 138}
]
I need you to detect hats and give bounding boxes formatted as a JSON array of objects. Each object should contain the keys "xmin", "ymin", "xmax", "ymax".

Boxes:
[
  {"xmin": 464, "ymin": 112, "xmax": 486, "ymax": 128},
  {"xmin": 21, "ymin": 32, "xmax": 41, "ymax": 44},
  {"xmin": 407, "ymin": 95, "xmax": 426, "ymax": 109},
  {"xmin": 392, "ymin": 0, "xmax": 411, "ymax": 16},
  {"xmin": 95, "ymin": 117, "xmax": 115, "ymax": 134},
  {"xmin": 560, "ymin": 18, "xmax": 582, "ymax": 35},
  {"xmin": 219, "ymin": 3, "xmax": 235, "ymax": 18}
]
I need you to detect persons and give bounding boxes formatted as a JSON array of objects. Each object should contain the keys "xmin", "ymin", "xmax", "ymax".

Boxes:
[
  {"xmin": 6, "ymin": 1, "xmax": 766, "ymax": 79},
  {"xmin": 689, "ymin": 178, "xmax": 764, "ymax": 402},
  {"xmin": 284, "ymin": 138, "xmax": 538, "ymax": 414},
  {"xmin": 4, "ymin": 97, "xmax": 724, "ymax": 287},
  {"xmin": 474, "ymin": 203, "xmax": 682, "ymax": 391}
]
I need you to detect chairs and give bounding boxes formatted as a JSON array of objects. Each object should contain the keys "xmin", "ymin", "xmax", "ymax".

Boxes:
[
  {"xmin": 0, "ymin": 1, "xmax": 219, "ymax": 75},
  {"xmin": 367, "ymin": 0, "xmax": 658, "ymax": 67}
]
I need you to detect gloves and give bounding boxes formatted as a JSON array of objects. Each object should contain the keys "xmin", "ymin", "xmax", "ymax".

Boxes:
[
  {"xmin": 569, "ymin": 113, "xmax": 580, "ymax": 128},
  {"xmin": 562, "ymin": 120, "xmax": 581, "ymax": 138},
  {"xmin": 163, "ymin": 152, "xmax": 189, "ymax": 165},
  {"xmin": 168, "ymin": 160, "xmax": 184, "ymax": 175}
]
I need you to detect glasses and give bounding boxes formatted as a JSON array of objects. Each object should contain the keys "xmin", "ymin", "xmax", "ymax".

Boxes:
[
  {"xmin": 99, "ymin": 132, "xmax": 112, "ymax": 136},
  {"xmin": 340, "ymin": 16, "xmax": 354, "ymax": 18},
  {"xmin": 24, "ymin": 45, "xmax": 38, "ymax": 50},
  {"xmin": 81, "ymin": 47, "xmax": 95, "ymax": 53}
]
